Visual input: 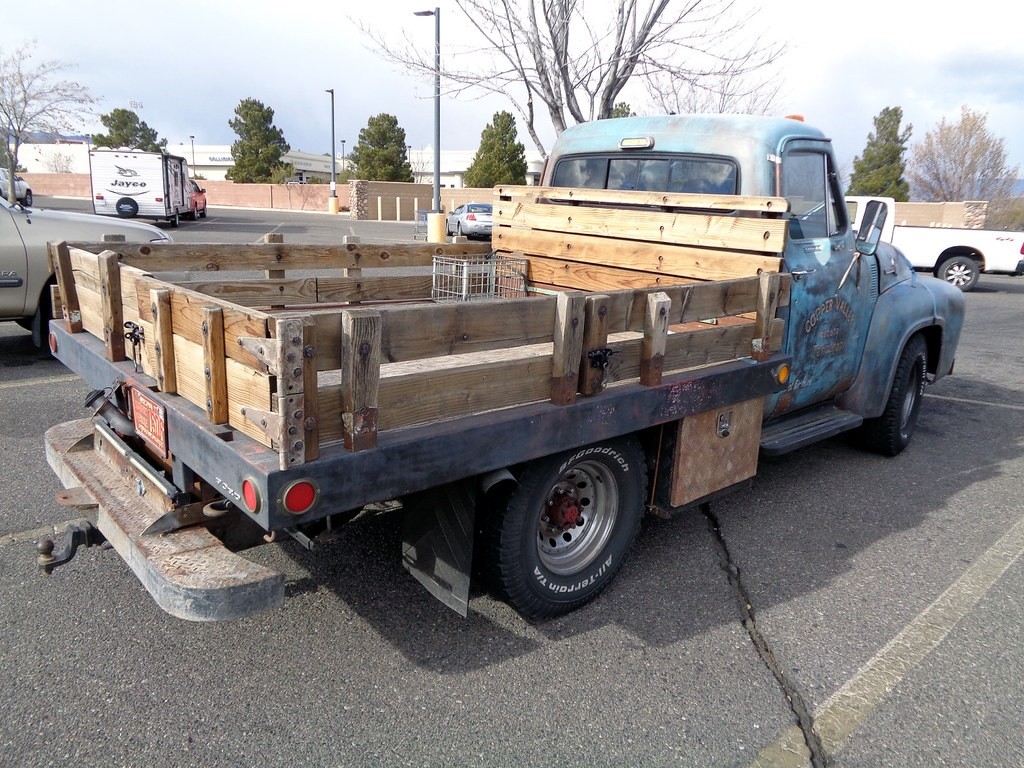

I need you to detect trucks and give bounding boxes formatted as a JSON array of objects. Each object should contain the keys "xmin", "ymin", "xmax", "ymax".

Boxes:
[{"xmin": 88, "ymin": 148, "xmax": 207, "ymax": 228}]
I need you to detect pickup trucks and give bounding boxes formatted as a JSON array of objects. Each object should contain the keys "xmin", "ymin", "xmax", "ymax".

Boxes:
[
  {"xmin": 38, "ymin": 111, "xmax": 966, "ymax": 623},
  {"xmin": 795, "ymin": 196, "xmax": 1024, "ymax": 292}
]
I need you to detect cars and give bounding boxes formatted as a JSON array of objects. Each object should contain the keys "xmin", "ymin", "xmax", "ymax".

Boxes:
[
  {"xmin": 444, "ymin": 203, "xmax": 492, "ymax": 241},
  {"xmin": 0, "ymin": 196, "xmax": 190, "ymax": 359},
  {"xmin": 0, "ymin": 168, "xmax": 33, "ymax": 207}
]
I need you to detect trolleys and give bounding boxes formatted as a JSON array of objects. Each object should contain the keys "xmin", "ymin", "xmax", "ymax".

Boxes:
[{"xmin": 413, "ymin": 209, "xmax": 431, "ymax": 242}]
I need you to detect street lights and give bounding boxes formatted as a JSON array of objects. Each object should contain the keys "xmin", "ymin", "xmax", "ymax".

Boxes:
[
  {"xmin": 190, "ymin": 136, "xmax": 196, "ymax": 180},
  {"xmin": 325, "ymin": 89, "xmax": 340, "ymax": 214},
  {"xmin": 412, "ymin": 6, "xmax": 446, "ymax": 244},
  {"xmin": 407, "ymin": 146, "xmax": 411, "ymax": 165},
  {"xmin": 341, "ymin": 139, "xmax": 345, "ymax": 172}
]
